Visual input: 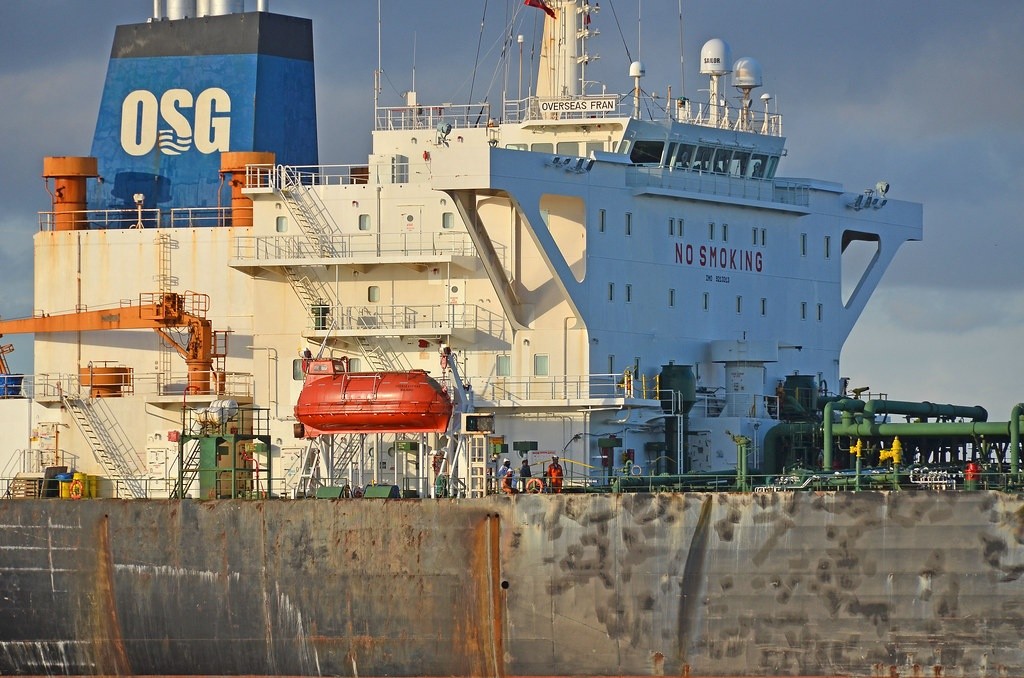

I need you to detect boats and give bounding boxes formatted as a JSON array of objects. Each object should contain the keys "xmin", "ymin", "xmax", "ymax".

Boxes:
[
  {"xmin": 293, "ymin": 353, "xmax": 453, "ymax": 438},
  {"xmin": 0, "ymin": 0, "xmax": 1024, "ymax": 678}
]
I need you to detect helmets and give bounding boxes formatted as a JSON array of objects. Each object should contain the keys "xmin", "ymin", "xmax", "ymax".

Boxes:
[
  {"xmin": 552, "ymin": 455, "xmax": 559, "ymax": 459},
  {"xmin": 507, "ymin": 469, "xmax": 513, "ymax": 472},
  {"xmin": 504, "ymin": 458, "xmax": 509, "ymax": 462}
]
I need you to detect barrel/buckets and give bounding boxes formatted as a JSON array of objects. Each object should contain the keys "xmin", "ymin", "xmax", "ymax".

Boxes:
[
  {"xmin": 58, "ymin": 472, "xmax": 97, "ymax": 499},
  {"xmin": 0, "ymin": 374, "xmax": 24, "ymax": 395}
]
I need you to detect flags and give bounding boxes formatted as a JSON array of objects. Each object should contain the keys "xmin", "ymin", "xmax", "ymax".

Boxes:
[{"xmin": 523, "ymin": 0, "xmax": 555, "ymax": 19}]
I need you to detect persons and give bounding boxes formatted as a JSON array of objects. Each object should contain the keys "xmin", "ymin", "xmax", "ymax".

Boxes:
[{"xmin": 497, "ymin": 456, "xmax": 566, "ymax": 495}]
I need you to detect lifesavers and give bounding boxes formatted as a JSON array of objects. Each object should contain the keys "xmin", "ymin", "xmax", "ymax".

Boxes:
[
  {"xmin": 631, "ymin": 464, "xmax": 642, "ymax": 475},
  {"xmin": 69, "ymin": 480, "xmax": 84, "ymax": 500},
  {"xmin": 525, "ymin": 477, "xmax": 544, "ymax": 494}
]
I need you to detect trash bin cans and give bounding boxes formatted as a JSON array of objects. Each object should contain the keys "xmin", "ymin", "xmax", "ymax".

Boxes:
[{"xmin": 59, "ymin": 472, "xmax": 97, "ymax": 498}]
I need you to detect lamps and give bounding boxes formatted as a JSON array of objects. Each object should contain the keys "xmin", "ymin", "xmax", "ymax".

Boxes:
[
  {"xmin": 846, "ymin": 181, "xmax": 890, "ymax": 211},
  {"xmin": 555, "ymin": 157, "xmax": 572, "ymax": 168},
  {"xmin": 544, "ymin": 156, "xmax": 560, "ymax": 167},
  {"xmin": 565, "ymin": 158, "xmax": 584, "ymax": 173},
  {"xmin": 579, "ymin": 159, "xmax": 595, "ymax": 172}
]
